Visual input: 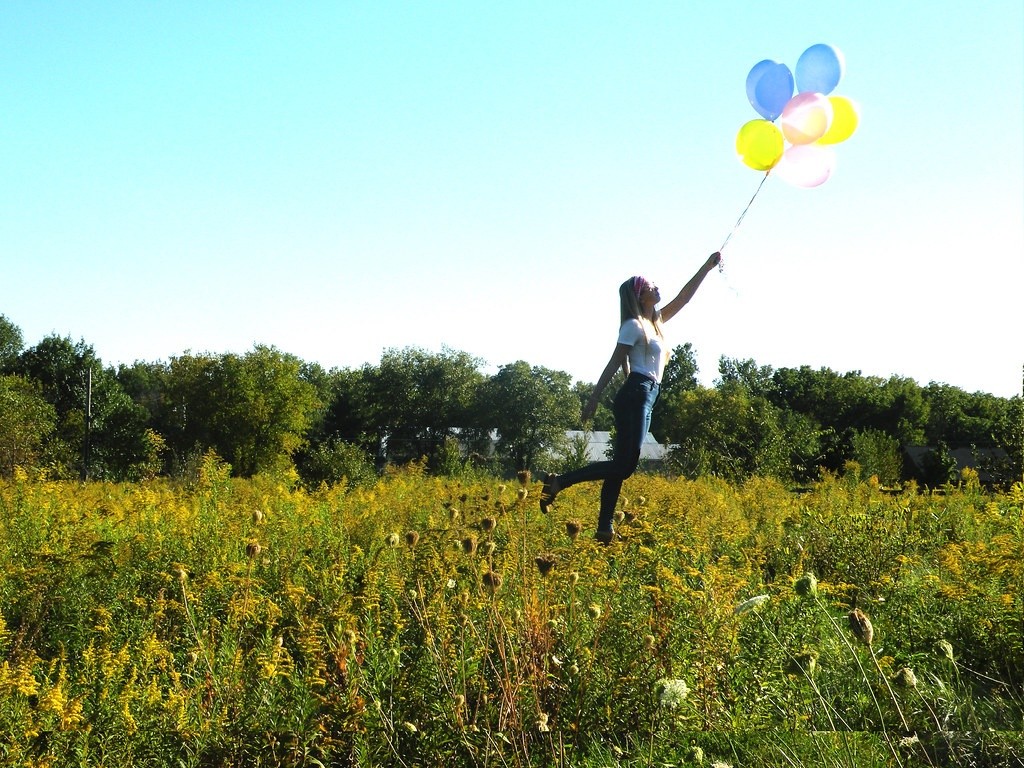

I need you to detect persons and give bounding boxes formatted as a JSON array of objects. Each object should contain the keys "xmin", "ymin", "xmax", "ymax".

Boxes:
[{"xmin": 540, "ymin": 251, "xmax": 722, "ymax": 547}]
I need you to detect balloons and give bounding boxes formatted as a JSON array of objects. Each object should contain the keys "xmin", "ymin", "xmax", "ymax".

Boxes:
[
  {"xmin": 782, "ymin": 91, "xmax": 833, "ymax": 145},
  {"xmin": 795, "ymin": 43, "xmax": 840, "ymax": 96},
  {"xmin": 744, "ymin": 59, "xmax": 794, "ymax": 120},
  {"xmin": 736, "ymin": 119, "xmax": 784, "ymax": 171},
  {"xmin": 812, "ymin": 96, "xmax": 858, "ymax": 146},
  {"xmin": 783, "ymin": 144, "xmax": 831, "ymax": 187},
  {"xmin": 755, "ymin": 62, "xmax": 794, "ymax": 113}
]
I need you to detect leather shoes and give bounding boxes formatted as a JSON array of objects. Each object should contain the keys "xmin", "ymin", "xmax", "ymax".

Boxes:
[
  {"xmin": 593, "ymin": 533, "xmax": 623, "ymax": 546},
  {"xmin": 540, "ymin": 473, "xmax": 558, "ymax": 514}
]
